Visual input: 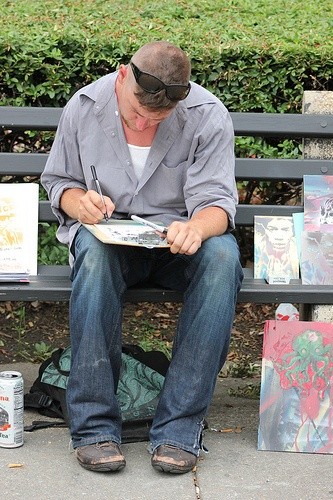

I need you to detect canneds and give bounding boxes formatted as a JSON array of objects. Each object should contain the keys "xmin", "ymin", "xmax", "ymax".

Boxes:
[{"xmin": 0, "ymin": 370, "xmax": 25, "ymax": 449}]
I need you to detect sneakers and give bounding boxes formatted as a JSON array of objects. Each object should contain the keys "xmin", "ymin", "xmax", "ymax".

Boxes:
[
  {"xmin": 74, "ymin": 441, "xmax": 125, "ymax": 471},
  {"xmin": 152, "ymin": 445, "xmax": 193, "ymax": 476}
]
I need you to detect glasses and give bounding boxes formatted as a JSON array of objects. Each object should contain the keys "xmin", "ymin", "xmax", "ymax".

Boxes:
[{"xmin": 131, "ymin": 62, "xmax": 191, "ymax": 101}]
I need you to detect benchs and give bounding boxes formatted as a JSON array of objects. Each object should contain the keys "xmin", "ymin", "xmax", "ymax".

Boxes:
[{"xmin": 0, "ymin": 107, "xmax": 332, "ymax": 322}]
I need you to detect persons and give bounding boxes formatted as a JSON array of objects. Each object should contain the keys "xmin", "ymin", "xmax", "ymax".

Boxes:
[{"xmin": 41, "ymin": 42, "xmax": 244, "ymax": 474}]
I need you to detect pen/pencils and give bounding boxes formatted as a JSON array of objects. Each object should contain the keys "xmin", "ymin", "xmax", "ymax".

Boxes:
[
  {"xmin": 131, "ymin": 214, "xmax": 168, "ymax": 236},
  {"xmin": 90, "ymin": 164, "xmax": 109, "ymax": 224}
]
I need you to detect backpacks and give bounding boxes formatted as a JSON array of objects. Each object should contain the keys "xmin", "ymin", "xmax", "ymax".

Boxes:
[{"xmin": 23, "ymin": 343, "xmax": 208, "ymax": 446}]
